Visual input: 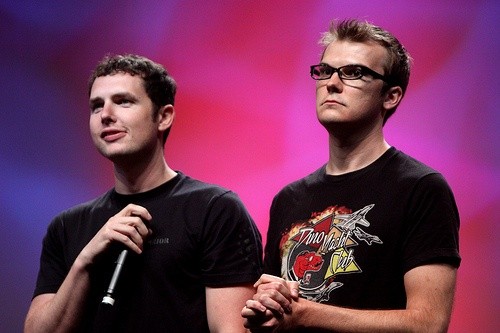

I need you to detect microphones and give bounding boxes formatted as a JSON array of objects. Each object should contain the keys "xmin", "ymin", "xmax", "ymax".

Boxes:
[{"xmin": 101, "ymin": 213, "xmax": 149, "ymax": 308}]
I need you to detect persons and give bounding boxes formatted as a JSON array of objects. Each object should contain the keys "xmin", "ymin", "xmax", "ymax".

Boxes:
[
  {"xmin": 23, "ymin": 55, "xmax": 264, "ymax": 333},
  {"xmin": 240, "ymin": 19, "xmax": 462, "ymax": 333}
]
